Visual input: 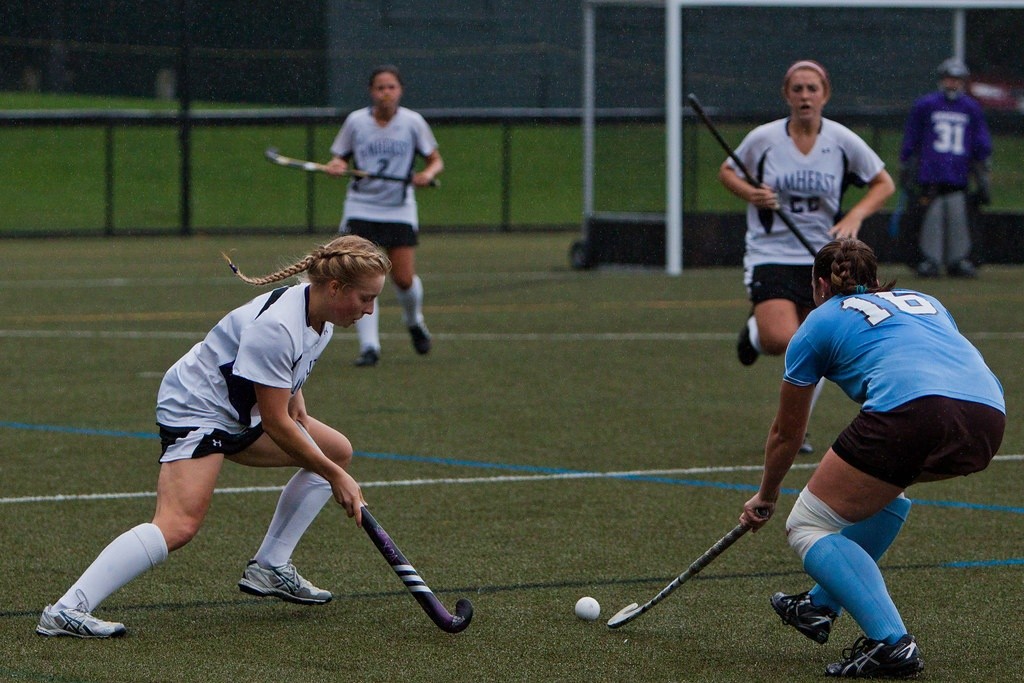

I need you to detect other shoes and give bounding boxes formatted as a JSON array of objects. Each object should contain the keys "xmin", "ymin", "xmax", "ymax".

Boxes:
[
  {"xmin": 798, "ymin": 431, "xmax": 814, "ymax": 453},
  {"xmin": 737, "ymin": 308, "xmax": 761, "ymax": 368},
  {"xmin": 406, "ymin": 318, "xmax": 433, "ymax": 354},
  {"xmin": 354, "ymin": 347, "xmax": 380, "ymax": 365}
]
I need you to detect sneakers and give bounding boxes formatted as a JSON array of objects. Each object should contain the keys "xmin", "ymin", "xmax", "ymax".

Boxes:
[
  {"xmin": 770, "ymin": 589, "xmax": 834, "ymax": 643},
  {"xmin": 824, "ymin": 633, "xmax": 926, "ymax": 676},
  {"xmin": 237, "ymin": 558, "xmax": 333, "ymax": 603},
  {"xmin": 37, "ymin": 602, "xmax": 127, "ymax": 640}
]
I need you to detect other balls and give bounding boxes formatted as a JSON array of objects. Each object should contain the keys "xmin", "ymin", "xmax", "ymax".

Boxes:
[{"xmin": 575, "ymin": 596, "xmax": 601, "ymax": 621}]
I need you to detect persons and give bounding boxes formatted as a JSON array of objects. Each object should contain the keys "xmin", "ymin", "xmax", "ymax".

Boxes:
[
  {"xmin": 717, "ymin": 58, "xmax": 899, "ymax": 456},
  {"xmin": 738, "ymin": 238, "xmax": 1005, "ymax": 675},
  {"xmin": 34, "ymin": 236, "xmax": 391, "ymax": 639},
  {"xmin": 331, "ymin": 62, "xmax": 447, "ymax": 368},
  {"xmin": 905, "ymin": 56, "xmax": 1015, "ymax": 278}
]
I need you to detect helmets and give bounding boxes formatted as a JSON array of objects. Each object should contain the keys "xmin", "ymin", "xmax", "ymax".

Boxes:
[{"xmin": 937, "ymin": 59, "xmax": 970, "ymax": 99}]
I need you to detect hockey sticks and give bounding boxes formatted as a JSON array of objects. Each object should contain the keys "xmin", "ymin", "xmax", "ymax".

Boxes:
[
  {"xmin": 294, "ymin": 420, "xmax": 474, "ymax": 634},
  {"xmin": 687, "ymin": 91, "xmax": 819, "ymax": 258},
  {"xmin": 607, "ymin": 507, "xmax": 772, "ymax": 629},
  {"xmin": 263, "ymin": 146, "xmax": 442, "ymax": 188}
]
